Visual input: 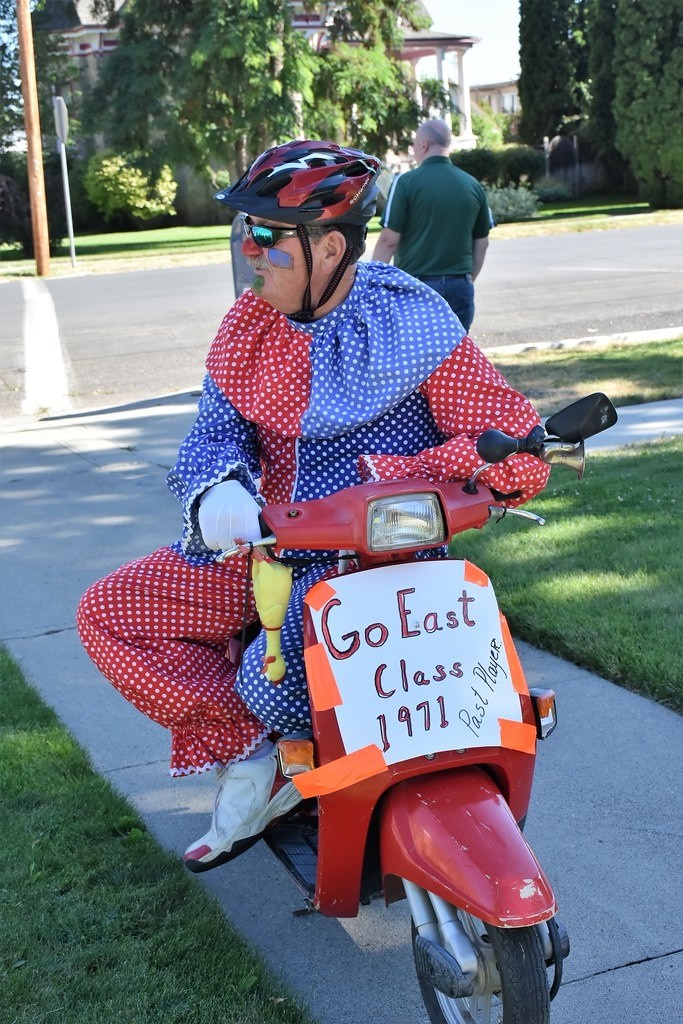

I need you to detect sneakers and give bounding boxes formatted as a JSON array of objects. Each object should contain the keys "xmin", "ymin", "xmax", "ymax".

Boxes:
[{"xmin": 183, "ymin": 742, "xmax": 304, "ymax": 873}]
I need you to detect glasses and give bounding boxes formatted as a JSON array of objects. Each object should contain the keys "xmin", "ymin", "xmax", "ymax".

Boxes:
[{"xmin": 243, "ymin": 215, "xmax": 298, "ymax": 249}]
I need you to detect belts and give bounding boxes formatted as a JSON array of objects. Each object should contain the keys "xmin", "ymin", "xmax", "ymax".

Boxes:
[{"xmin": 412, "ymin": 273, "xmax": 465, "ymax": 282}]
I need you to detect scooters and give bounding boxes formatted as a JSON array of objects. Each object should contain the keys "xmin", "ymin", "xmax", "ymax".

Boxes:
[{"xmin": 216, "ymin": 392, "xmax": 621, "ymax": 1020}]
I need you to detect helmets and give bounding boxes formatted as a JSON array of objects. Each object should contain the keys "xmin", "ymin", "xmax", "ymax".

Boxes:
[{"xmin": 213, "ymin": 139, "xmax": 383, "ymax": 227}]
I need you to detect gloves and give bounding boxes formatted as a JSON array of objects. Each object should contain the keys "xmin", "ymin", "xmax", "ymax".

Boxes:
[
  {"xmin": 337, "ymin": 474, "xmax": 375, "ymax": 575},
  {"xmin": 198, "ymin": 480, "xmax": 264, "ymax": 552}
]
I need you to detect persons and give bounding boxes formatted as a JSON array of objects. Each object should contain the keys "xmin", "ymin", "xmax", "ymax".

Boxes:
[
  {"xmin": 78, "ymin": 140, "xmax": 551, "ymax": 871},
  {"xmin": 369, "ymin": 121, "xmax": 491, "ymax": 334}
]
